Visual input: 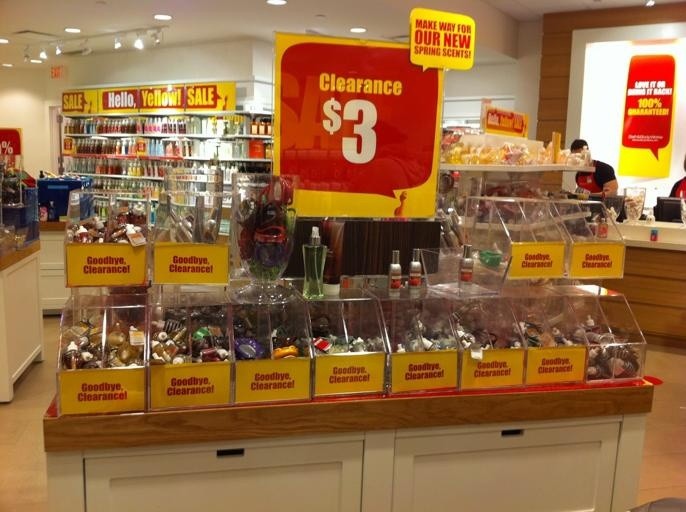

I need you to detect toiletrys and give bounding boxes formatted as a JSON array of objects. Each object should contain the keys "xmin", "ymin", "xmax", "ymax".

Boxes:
[
  {"xmin": 408, "ymin": 247, "xmax": 423, "ymax": 290},
  {"xmin": 389, "ymin": 249, "xmax": 402, "ymax": 291}
]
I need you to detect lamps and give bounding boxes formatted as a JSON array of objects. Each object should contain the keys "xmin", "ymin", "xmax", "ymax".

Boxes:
[
  {"xmin": 55, "ymin": 40, "xmax": 64, "ymax": 55},
  {"xmin": 23, "ymin": 45, "xmax": 33, "ymax": 64},
  {"xmin": 115, "ymin": 34, "xmax": 122, "ymax": 49},
  {"xmin": 81, "ymin": 39, "xmax": 92, "ymax": 56},
  {"xmin": 133, "ymin": 32, "xmax": 143, "ymax": 50}
]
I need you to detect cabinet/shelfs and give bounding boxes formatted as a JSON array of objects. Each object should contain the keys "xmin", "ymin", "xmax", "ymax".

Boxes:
[
  {"xmin": 61, "ymin": 110, "xmax": 275, "ymax": 239},
  {"xmin": 437, "ymin": 162, "xmax": 597, "ymax": 254},
  {"xmin": 0, "ymin": 240, "xmax": 45, "ymax": 402},
  {"xmin": 42, "ymin": 378, "xmax": 654, "ymax": 511}
]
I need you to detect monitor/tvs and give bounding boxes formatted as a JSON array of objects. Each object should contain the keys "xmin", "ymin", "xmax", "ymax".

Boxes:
[
  {"xmin": 566, "ymin": 193, "xmax": 606, "ymax": 223},
  {"xmin": 653, "ymin": 197, "xmax": 686, "ymax": 222}
]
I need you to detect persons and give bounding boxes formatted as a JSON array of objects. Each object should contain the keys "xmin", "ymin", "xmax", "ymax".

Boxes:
[
  {"xmin": 666, "ymin": 154, "xmax": 686, "ymax": 201},
  {"xmin": 570, "ymin": 139, "xmax": 619, "ymax": 200}
]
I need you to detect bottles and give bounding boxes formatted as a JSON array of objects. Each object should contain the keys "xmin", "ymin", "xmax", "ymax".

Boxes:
[
  {"xmin": 387, "ymin": 250, "xmax": 402, "ymax": 296},
  {"xmin": 408, "ymin": 248, "xmax": 424, "ymax": 295},
  {"xmin": 64, "ymin": 117, "xmax": 272, "ymax": 233},
  {"xmin": 302, "ymin": 226, "xmax": 328, "ymax": 299}
]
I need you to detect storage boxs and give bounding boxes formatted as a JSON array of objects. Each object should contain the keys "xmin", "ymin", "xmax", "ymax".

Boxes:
[
  {"xmin": 54, "ymin": 189, "xmax": 648, "ymax": 421},
  {"xmin": 37, "ymin": 176, "xmax": 93, "ymax": 222}
]
